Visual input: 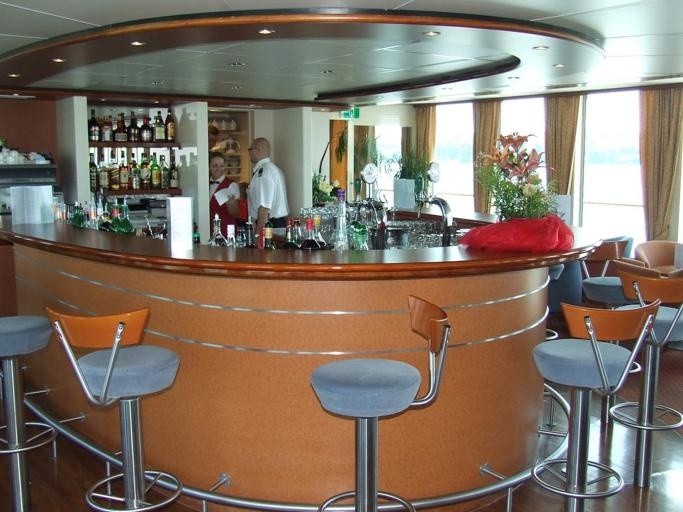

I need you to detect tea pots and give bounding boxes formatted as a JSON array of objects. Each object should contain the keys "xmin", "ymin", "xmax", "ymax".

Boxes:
[{"xmin": 223, "ymin": 136, "xmax": 240, "ymax": 153}]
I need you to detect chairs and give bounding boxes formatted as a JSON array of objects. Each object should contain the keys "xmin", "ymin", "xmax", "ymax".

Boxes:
[
  {"xmin": 43, "ymin": 302, "xmax": 180, "ymax": 512},
  {"xmin": 309, "ymin": 292, "xmax": 452, "ymax": 511}
]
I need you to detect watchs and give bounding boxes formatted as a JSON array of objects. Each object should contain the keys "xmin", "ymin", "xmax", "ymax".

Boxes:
[{"xmin": 254, "ymin": 233, "xmax": 261, "ymax": 239}]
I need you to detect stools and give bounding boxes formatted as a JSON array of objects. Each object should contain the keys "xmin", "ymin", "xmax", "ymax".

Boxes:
[{"xmin": 0, "ymin": 315, "xmax": 58, "ymax": 512}]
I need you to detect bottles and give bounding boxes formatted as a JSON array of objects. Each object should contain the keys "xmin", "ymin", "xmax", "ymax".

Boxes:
[
  {"xmin": 87, "ymin": 105, "xmax": 175, "ymax": 141},
  {"xmin": 193, "ymin": 213, "xmax": 326, "ymax": 250},
  {"xmin": 54, "ymin": 192, "xmax": 134, "ymax": 234},
  {"xmin": 209, "ymin": 117, "xmax": 237, "ymax": 130},
  {"xmin": 88, "ymin": 149, "xmax": 179, "ymax": 190},
  {"xmin": 326, "ymin": 188, "xmax": 368, "ymax": 252}
]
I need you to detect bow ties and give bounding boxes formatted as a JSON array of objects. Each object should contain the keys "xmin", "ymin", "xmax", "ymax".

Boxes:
[{"xmin": 209, "ymin": 180, "xmax": 220, "ymax": 185}]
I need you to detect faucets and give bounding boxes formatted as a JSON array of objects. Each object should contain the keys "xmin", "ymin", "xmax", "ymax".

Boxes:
[{"xmin": 416, "ymin": 194, "xmax": 453, "ymax": 247}]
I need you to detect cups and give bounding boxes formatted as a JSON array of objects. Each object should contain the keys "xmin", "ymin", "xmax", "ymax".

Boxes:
[
  {"xmin": 412, "ymin": 228, "xmax": 459, "ymax": 247},
  {"xmin": 299, "ymin": 205, "xmax": 358, "ymax": 229},
  {"xmin": 0, "ymin": 146, "xmax": 44, "ymax": 164}
]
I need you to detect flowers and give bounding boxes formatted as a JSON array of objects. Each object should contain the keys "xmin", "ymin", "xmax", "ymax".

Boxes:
[
  {"xmin": 470, "ymin": 130, "xmax": 564, "ymax": 220},
  {"xmin": 312, "ymin": 172, "xmax": 339, "ymax": 202}
]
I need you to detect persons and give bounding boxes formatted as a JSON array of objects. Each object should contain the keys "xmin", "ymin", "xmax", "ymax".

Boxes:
[
  {"xmin": 246, "ymin": 136, "xmax": 292, "ymax": 250},
  {"xmin": 209, "ymin": 152, "xmax": 242, "ymax": 239}
]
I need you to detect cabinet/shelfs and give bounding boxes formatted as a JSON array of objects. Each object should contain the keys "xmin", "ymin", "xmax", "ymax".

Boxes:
[
  {"xmin": 207, "ymin": 105, "xmax": 251, "ymax": 184},
  {"xmin": 87, "ymin": 140, "xmax": 182, "ymax": 196}
]
